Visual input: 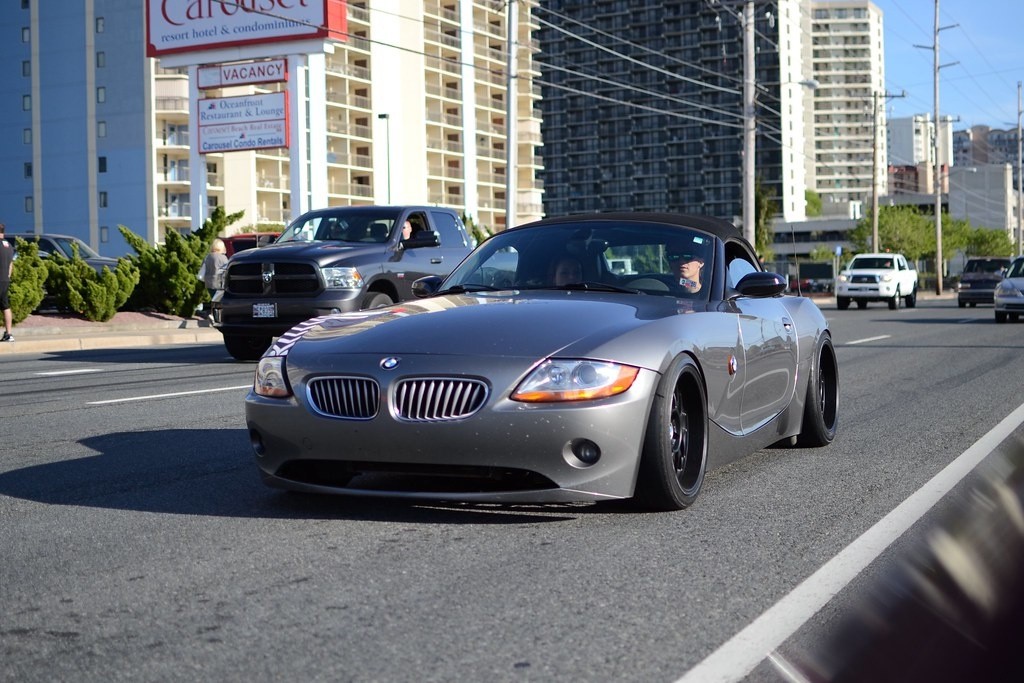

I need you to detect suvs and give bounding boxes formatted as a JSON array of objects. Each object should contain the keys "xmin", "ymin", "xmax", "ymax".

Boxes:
[{"xmin": 214, "ymin": 207, "xmax": 475, "ymax": 361}]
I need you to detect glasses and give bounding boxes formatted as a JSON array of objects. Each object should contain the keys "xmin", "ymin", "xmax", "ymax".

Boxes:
[
  {"xmin": 402, "ymin": 226, "xmax": 406, "ymax": 229},
  {"xmin": 557, "ymin": 268, "xmax": 581, "ymax": 279},
  {"xmin": 665, "ymin": 252, "xmax": 696, "ymax": 262}
]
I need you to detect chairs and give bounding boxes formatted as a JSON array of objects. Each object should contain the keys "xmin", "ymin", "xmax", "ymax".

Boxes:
[
  {"xmin": 320, "ymin": 221, "xmax": 354, "ymax": 241},
  {"xmin": 544, "ymin": 253, "xmax": 597, "ymax": 288},
  {"xmin": 366, "ymin": 223, "xmax": 388, "ymax": 243}
]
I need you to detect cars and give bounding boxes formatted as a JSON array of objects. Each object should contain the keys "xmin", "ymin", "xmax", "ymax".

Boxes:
[
  {"xmin": 245, "ymin": 211, "xmax": 840, "ymax": 513},
  {"xmin": 994, "ymin": 257, "xmax": 1024, "ymax": 323},
  {"xmin": 958, "ymin": 258, "xmax": 1010, "ymax": 308}
]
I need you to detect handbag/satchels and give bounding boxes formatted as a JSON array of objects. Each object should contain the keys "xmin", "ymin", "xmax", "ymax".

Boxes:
[{"xmin": 197, "ymin": 254, "xmax": 207, "ymax": 282}]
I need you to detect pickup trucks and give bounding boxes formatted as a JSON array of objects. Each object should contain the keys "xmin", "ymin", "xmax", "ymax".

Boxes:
[{"xmin": 836, "ymin": 253, "xmax": 918, "ymax": 311}]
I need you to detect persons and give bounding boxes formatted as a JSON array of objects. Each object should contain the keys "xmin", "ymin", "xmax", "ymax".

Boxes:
[
  {"xmin": 400, "ymin": 220, "xmax": 412, "ymax": 241},
  {"xmin": 666, "ymin": 239, "xmax": 710, "ymax": 300},
  {"xmin": 0, "ymin": 224, "xmax": 13, "ymax": 342},
  {"xmin": 197, "ymin": 239, "xmax": 229, "ymax": 327},
  {"xmin": 975, "ymin": 265, "xmax": 984, "ymax": 272},
  {"xmin": 549, "ymin": 253, "xmax": 585, "ymax": 286},
  {"xmin": 883, "ymin": 261, "xmax": 891, "ymax": 267},
  {"xmin": 994, "ymin": 264, "xmax": 1007, "ymax": 274}
]
